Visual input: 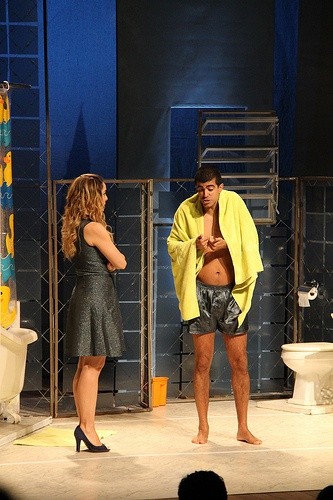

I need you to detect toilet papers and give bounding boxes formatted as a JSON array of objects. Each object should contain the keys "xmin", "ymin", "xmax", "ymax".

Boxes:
[{"xmin": 297, "ymin": 286, "xmax": 317, "ymax": 308}]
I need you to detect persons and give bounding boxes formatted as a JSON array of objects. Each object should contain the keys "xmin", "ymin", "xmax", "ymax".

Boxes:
[
  {"xmin": 178, "ymin": 469, "xmax": 229, "ymax": 499},
  {"xmin": 61, "ymin": 174, "xmax": 129, "ymax": 453},
  {"xmin": 165, "ymin": 167, "xmax": 265, "ymax": 444}
]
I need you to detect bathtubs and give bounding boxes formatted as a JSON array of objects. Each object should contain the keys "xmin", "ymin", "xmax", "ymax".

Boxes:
[{"xmin": 0, "ymin": 323, "xmax": 38, "ymax": 426}]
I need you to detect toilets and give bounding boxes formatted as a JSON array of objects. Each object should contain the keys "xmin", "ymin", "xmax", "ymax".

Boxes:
[{"xmin": 279, "ymin": 340, "xmax": 333, "ymax": 406}]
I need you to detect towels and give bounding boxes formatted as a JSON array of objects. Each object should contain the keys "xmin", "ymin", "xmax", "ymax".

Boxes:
[{"xmin": 166, "ymin": 188, "xmax": 265, "ymax": 329}]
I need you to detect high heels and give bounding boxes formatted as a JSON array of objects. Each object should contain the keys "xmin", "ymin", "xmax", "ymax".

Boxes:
[{"xmin": 74, "ymin": 426, "xmax": 108, "ymax": 452}]
